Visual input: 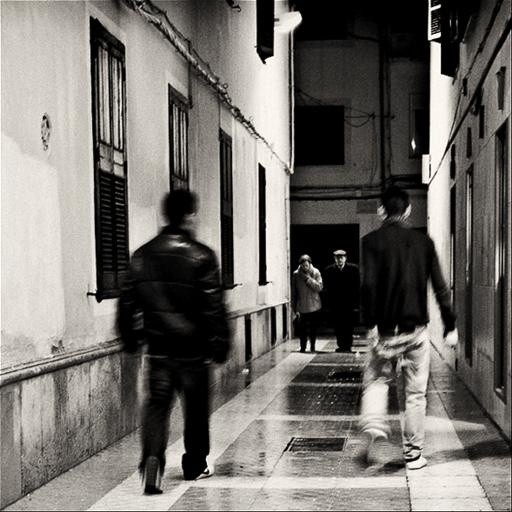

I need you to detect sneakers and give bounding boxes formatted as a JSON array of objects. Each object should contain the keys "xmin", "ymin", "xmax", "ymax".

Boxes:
[
  {"xmin": 144, "ymin": 457, "xmax": 162, "ymax": 494},
  {"xmin": 406, "ymin": 457, "xmax": 428, "ymax": 470},
  {"xmin": 194, "ymin": 464, "xmax": 214, "ymax": 479}
]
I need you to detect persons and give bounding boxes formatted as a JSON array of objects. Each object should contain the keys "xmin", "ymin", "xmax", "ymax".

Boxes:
[
  {"xmin": 354, "ymin": 185, "xmax": 459, "ymax": 471},
  {"xmin": 322, "ymin": 249, "xmax": 359, "ymax": 352},
  {"xmin": 291, "ymin": 254, "xmax": 324, "ymax": 353},
  {"xmin": 114, "ymin": 187, "xmax": 231, "ymax": 493}
]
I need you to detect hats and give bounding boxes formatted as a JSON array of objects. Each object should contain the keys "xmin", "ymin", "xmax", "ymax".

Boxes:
[{"xmin": 333, "ymin": 249, "xmax": 347, "ymax": 256}]
